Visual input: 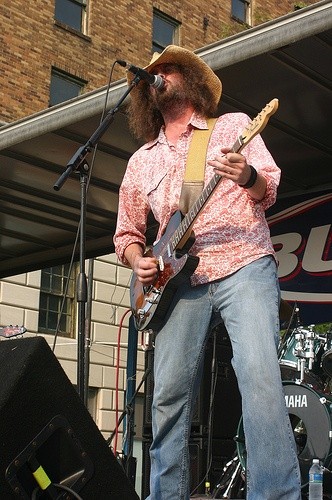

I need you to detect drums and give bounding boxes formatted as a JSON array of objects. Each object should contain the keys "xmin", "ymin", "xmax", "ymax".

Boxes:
[
  {"xmin": 278, "ymin": 329, "xmax": 332, "ymax": 390},
  {"xmin": 234, "ymin": 380, "xmax": 332, "ymax": 493}
]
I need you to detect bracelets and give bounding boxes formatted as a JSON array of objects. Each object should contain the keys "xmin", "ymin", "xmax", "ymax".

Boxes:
[{"xmin": 238, "ymin": 165, "xmax": 257, "ymax": 189}]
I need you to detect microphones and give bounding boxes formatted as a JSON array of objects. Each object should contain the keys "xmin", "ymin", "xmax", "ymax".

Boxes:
[{"xmin": 117, "ymin": 60, "xmax": 165, "ymax": 91}]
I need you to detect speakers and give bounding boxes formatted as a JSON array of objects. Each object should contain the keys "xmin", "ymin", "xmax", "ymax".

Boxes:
[
  {"xmin": 141, "ymin": 343, "xmax": 243, "ymax": 500},
  {"xmin": 0, "ymin": 336, "xmax": 141, "ymax": 500}
]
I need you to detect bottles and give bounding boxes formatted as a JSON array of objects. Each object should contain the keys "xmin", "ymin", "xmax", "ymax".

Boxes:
[{"xmin": 309, "ymin": 459, "xmax": 323, "ymax": 500}]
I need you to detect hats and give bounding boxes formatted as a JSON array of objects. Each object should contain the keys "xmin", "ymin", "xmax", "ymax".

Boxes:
[{"xmin": 125, "ymin": 44, "xmax": 222, "ymax": 112}]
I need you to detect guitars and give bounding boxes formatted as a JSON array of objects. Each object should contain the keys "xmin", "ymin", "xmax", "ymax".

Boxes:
[{"xmin": 130, "ymin": 98, "xmax": 279, "ymax": 332}]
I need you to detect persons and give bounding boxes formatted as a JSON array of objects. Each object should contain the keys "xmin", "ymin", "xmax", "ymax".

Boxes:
[{"xmin": 112, "ymin": 44, "xmax": 301, "ymax": 500}]
